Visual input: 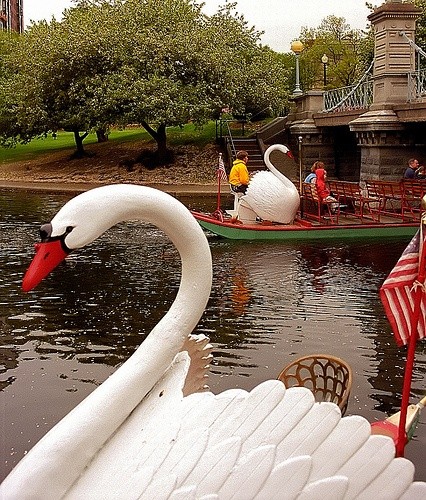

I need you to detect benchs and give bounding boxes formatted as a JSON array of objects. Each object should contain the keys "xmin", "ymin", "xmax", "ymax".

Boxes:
[{"xmin": 297, "ymin": 178, "xmax": 426, "ymax": 225}]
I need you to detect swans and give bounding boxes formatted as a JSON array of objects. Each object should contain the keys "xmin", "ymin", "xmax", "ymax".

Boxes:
[
  {"xmin": 239, "ymin": 144, "xmax": 302, "ymax": 224},
  {"xmin": 0, "ymin": 184, "xmax": 425, "ymax": 499}
]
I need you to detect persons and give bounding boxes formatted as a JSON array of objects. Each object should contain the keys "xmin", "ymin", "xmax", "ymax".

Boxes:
[
  {"xmin": 404, "ymin": 158, "xmax": 426, "ymax": 207},
  {"xmin": 228, "ymin": 150, "xmax": 264, "ymax": 223},
  {"xmin": 304, "ymin": 161, "xmax": 339, "ymax": 214}
]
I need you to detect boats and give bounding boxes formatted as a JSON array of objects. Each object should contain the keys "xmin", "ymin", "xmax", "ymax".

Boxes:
[
  {"xmin": 0, "ymin": 184, "xmax": 425, "ymax": 498},
  {"xmin": 191, "ymin": 143, "xmax": 426, "ymax": 243}
]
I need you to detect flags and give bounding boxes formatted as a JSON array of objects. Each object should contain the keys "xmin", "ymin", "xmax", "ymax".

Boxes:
[{"xmin": 379, "ymin": 226, "xmax": 426, "ymax": 347}]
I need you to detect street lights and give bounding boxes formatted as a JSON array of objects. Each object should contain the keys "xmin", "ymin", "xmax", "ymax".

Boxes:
[
  {"xmin": 290, "ymin": 40, "xmax": 308, "ymax": 99},
  {"xmin": 321, "ymin": 53, "xmax": 332, "ymax": 89}
]
